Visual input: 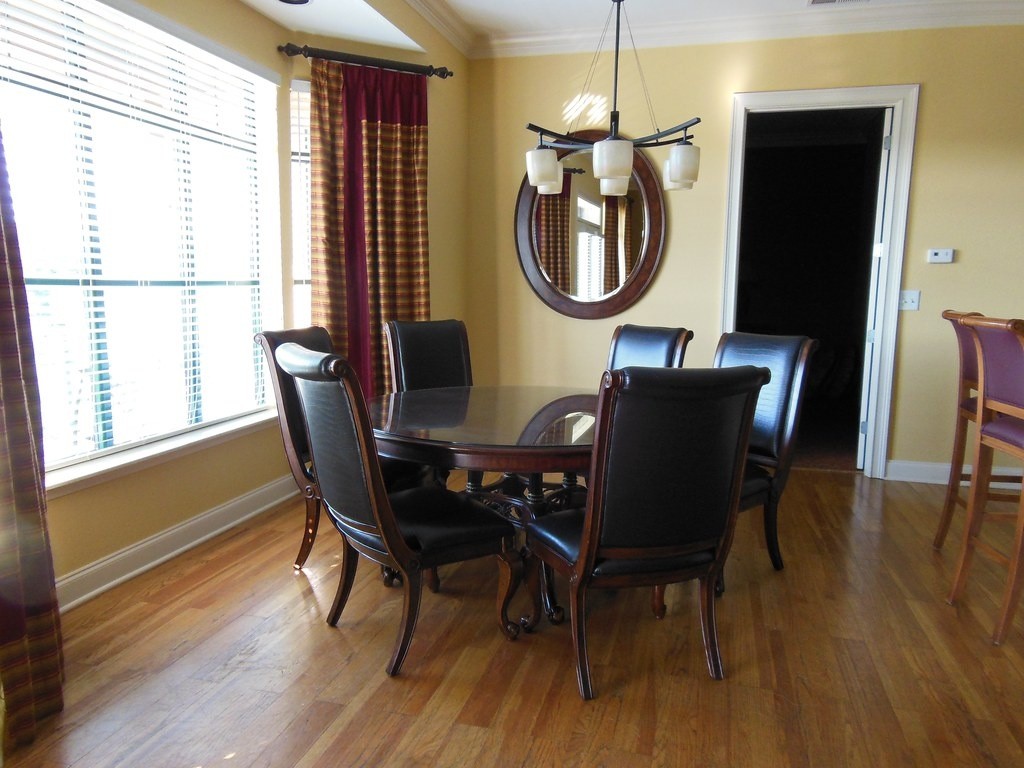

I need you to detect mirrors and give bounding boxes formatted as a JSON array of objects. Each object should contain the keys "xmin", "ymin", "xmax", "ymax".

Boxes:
[{"xmin": 514, "ymin": 130, "xmax": 666, "ymax": 320}]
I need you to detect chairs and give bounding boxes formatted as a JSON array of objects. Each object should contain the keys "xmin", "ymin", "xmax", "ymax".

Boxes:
[
  {"xmin": 932, "ymin": 310, "xmax": 1024, "ymax": 645},
  {"xmin": 254, "ymin": 319, "xmax": 816, "ymax": 700}
]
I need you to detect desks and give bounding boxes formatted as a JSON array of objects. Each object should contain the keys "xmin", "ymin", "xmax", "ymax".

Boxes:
[{"xmin": 365, "ymin": 386, "xmax": 595, "ymax": 631}]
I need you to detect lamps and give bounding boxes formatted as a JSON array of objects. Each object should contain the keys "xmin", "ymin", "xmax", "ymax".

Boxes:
[{"xmin": 526, "ymin": 0, "xmax": 701, "ymax": 195}]
